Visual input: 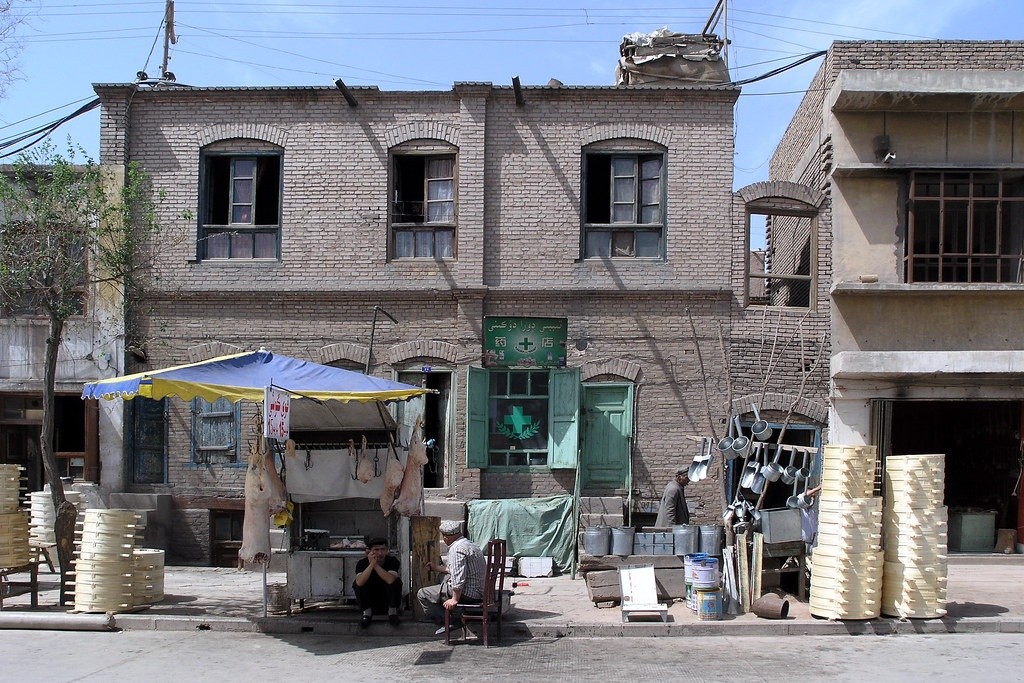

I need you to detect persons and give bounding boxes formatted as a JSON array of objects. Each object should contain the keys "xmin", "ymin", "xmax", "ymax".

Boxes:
[
  {"xmin": 654, "ymin": 467, "xmax": 691, "ymax": 527},
  {"xmin": 352, "ymin": 537, "xmax": 404, "ymax": 630},
  {"xmin": 417, "ymin": 520, "xmax": 491, "ymax": 635}
]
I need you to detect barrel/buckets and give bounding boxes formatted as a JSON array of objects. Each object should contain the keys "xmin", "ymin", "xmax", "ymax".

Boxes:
[
  {"xmin": 264, "ymin": 583, "xmax": 289, "ymax": 613},
  {"xmin": 671, "ymin": 523, "xmax": 725, "ymax": 621},
  {"xmin": 585, "ymin": 525, "xmax": 635, "ymax": 557},
  {"xmin": 751, "ymin": 597, "xmax": 790, "ymax": 619}
]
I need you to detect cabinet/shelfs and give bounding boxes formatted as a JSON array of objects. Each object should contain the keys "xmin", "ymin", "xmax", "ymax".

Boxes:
[{"xmin": 285, "ymin": 493, "xmax": 410, "ymax": 618}]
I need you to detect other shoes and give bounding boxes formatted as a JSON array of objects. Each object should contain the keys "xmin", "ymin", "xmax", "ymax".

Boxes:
[
  {"xmin": 435, "ymin": 621, "xmax": 464, "ymax": 634},
  {"xmin": 360, "ymin": 615, "xmax": 371, "ymax": 628},
  {"xmin": 388, "ymin": 614, "xmax": 401, "ymax": 625}
]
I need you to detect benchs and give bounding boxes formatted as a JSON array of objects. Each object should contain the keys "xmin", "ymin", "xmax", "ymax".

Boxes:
[{"xmin": 28, "ymin": 539, "xmax": 58, "ymax": 574}]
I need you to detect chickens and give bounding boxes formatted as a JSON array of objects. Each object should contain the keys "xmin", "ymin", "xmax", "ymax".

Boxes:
[{"xmin": 357, "ymin": 434, "xmax": 374, "ymax": 484}]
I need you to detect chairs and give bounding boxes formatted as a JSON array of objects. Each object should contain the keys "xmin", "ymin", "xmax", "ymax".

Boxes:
[{"xmin": 444, "ymin": 539, "xmax": 507, "ymax": 647}]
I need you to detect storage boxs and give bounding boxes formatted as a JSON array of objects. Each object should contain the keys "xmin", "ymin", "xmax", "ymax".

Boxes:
[{"xmin": 761, "ymin": 508, "xmax": 803, "ymax": 544}]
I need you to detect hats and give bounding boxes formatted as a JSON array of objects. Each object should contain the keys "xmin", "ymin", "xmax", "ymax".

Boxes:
[
  {"xmin": 674, "ymin": 466, "xmax": 689, "ymax": 476},
  {"xmin": 438, "ymin": 520, "xmax": 461, "ymax": 535}
]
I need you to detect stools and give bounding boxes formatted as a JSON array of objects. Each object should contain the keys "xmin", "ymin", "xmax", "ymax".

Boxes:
[{"xmin": 621, "ymin": 604, "xmax": 667, "ymax": 624}]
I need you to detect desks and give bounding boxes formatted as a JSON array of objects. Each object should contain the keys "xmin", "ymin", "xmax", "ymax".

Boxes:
[
  {"xmin": 948, "ymin": 511, "xmax": 997, "ymax": 552},
  {"xmin": 0, "ymin": 562, "xmax": 40, "ymax": 611}
]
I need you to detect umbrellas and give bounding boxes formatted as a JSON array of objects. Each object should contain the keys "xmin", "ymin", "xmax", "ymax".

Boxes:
[{"xmin": 81, "ymin": 347, "xmax": 440, "ymax": 618}]
